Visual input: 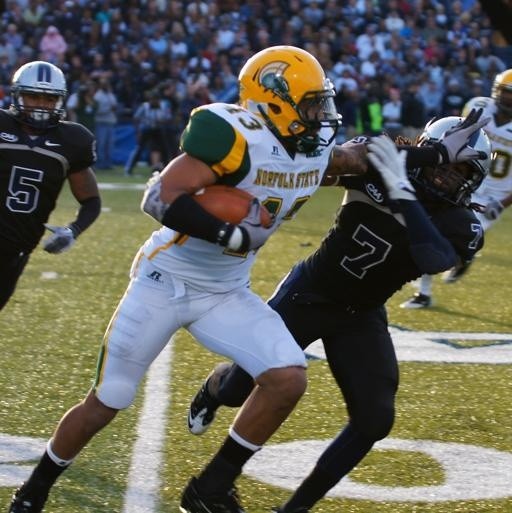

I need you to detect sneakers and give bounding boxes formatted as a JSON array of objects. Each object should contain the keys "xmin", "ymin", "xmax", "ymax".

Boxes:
[
  {"xmin": 443, "ymin": 260, "xmax": 472, "ymax": 282},
  {"xmin": 179, "ymin": 475, "xmax": 246, "ymax": 513},
  {"xmin": 400, "ymin": 292, "xmax": 432, "ymax": 308},
  {"xmin": 7, "ymin": 481, "xmax": 49, "ymax": 513},
  {"xmin": 187, "ymin": 364, "xmax": 229, "ymax": 435}
]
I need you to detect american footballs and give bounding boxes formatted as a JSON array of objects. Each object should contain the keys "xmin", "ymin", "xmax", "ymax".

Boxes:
[{"xmin": 192, "ymin": 186, "xmax": 273, "ymax": 230}]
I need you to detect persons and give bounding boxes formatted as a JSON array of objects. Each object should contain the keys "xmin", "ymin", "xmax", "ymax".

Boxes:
[
  {"xmin": 188, "ymin": 118, "xmax": 493, "ymax": 512},
  {"xmin": 8, "ymin": 44, "xmax": 493, "ymax": 512},
  {"xmin": 1, "ymin": 61, "xmax": 101, "ymax": 313},
  {"xmin": 397, "ymin": 69, "xmax": 512, "ymax": 309},
  {"xmin": 0, "ymin": 0, "xmax": 512, "ymax": 177}
]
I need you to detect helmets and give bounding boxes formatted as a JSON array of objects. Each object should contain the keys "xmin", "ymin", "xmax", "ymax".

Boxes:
[
  {"xmin": 415, "ymin": 114, "xmax": 492, "ymax": 204},
  {"xmin": 10, "ymin": 61, "xmax": 69, "ymax": 129},
  {"xmin": 491, "ymin": 68, "xmax": 512, "ymax": 110},
  {"xmin": 237, "ymin": 46, "xmax": 343, "ymax": 153}
]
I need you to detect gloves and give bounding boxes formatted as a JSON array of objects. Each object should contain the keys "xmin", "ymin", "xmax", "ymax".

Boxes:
[
  {"xmin": 423, "ymin": 108, "xmax": 492, "ymax": 163},
  {"xmin": 42, "ymin": 222, "xmax": 76, "ymax": 254},
  {"xmin": 484, "ymin": 199, "xmax": 504, "ymax": 220},
  {"xmin": 233, "ymin": 198, "xmax": 280, "ymax": 255},
  {"xmin": 365, "ymin": 135, "xmax": 419, "ymax": 214}
]
